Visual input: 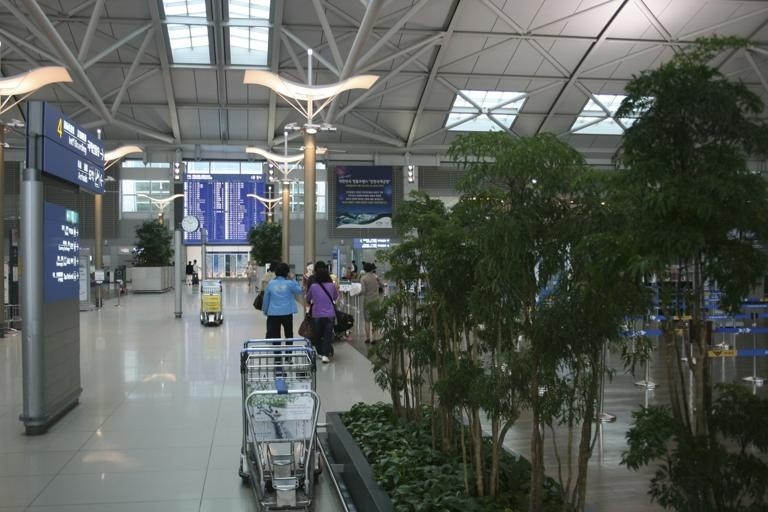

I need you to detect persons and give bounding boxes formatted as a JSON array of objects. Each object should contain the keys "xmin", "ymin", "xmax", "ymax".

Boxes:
[
  {"xmin": 186, "ymin": 260, "xmax": 201, "ymax": 285},
  {"xmin": 356, "ymin": 262, "xmax": 384, "ymax": 343},
  {"xmin": 261, "ymin": 260, "xmax": 339, "ymax": 364}
]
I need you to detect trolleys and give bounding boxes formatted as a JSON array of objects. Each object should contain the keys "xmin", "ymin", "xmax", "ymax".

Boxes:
[
  {"xmin": 238, "ymin": 338, "xmax": 322, "ymax": 512},
  {"xmin": 200, "ymin": 278, "xmax": 224, "ymax": 326}
]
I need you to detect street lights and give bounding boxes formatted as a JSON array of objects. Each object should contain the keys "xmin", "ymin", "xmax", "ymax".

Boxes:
[
  {"xmin": 242, "ymin": 48, "xmax": 379, "ymax": 269},
  {"xmin": 94, "ymin": 129, "xmax": 143, "ymax": 306}
]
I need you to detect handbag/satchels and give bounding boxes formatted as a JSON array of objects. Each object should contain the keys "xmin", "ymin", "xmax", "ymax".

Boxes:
[
  {"xmin": 297, "ymin": 313, "xmax": 315, "ymax": 340},
  {"xmin": 253, "ymin": 290, "xmax": 263, "ymax": 310},
  {"xmin": 335, "ymin": 311, "xmax": 354, "ymax": 334}
]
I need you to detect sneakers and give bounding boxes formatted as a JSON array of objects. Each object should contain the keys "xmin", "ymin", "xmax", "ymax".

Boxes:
[{"xmin": 317, "ymin": 353, "xmax": 333, "ymax": 362}]
